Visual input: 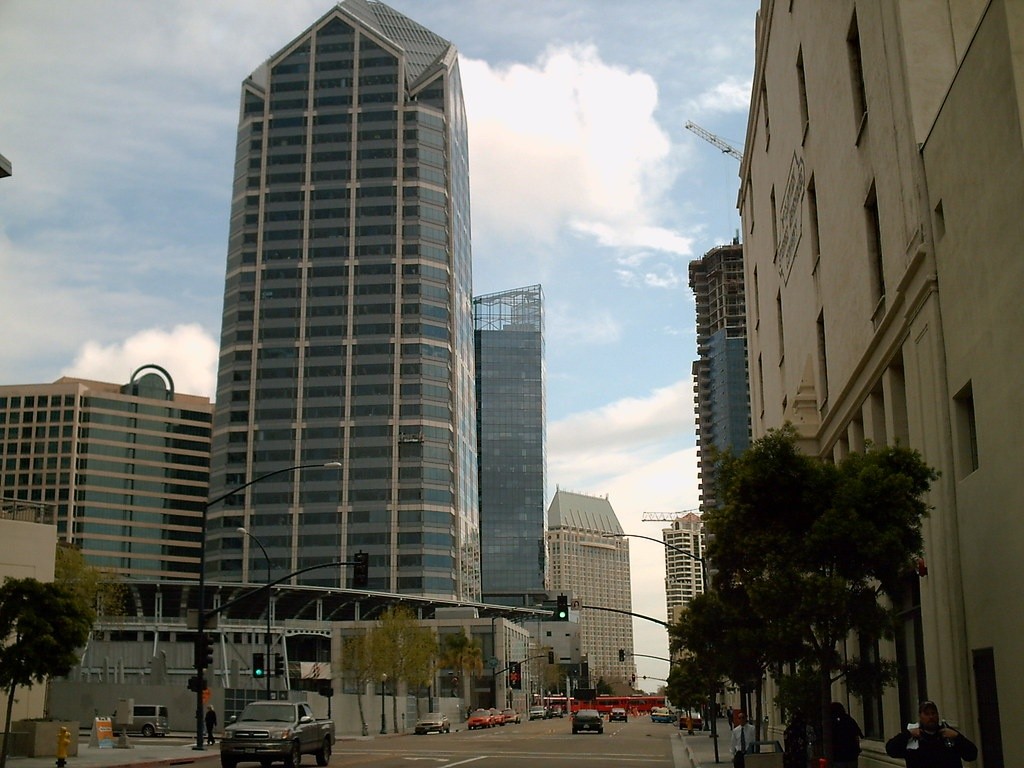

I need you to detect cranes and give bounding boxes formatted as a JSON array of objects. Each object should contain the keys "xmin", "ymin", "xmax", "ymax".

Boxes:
[
  {"xmin": 641, "ymin": 507, "xmax": 703, "ymax": 524},
  {"xmin": 685, "ymin": 120, "xmax": 743, "ymax": 163}
]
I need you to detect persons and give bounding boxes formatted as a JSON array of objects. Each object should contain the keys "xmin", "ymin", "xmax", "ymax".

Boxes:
[
  {"xmin": 205, "ymin": 706, "xmax": 217, "ymax": 745},
  {"xmin": 886, "ymin": 702, "xmax": 978, "ymax": 768},
  {"xmin": 727, "ymin": 707, "xmax": 736, "ymax": 730},
  {"xmin": 831, "ymin": 702, "xmax": 862, "ymax": 768},
  {"xmin": 731, "ymin": 713, "xmax": 755, "ymax": 768}
]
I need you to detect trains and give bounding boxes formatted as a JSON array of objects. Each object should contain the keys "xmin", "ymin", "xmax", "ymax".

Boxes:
[{"xmin": 536, "ymin": 697, "xmax": 670, "ymax": 712}]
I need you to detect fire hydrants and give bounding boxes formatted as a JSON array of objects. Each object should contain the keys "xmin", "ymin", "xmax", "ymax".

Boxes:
[{"xmin": 55, "ymin": 727, "xmax": 71, "ymax": 768}]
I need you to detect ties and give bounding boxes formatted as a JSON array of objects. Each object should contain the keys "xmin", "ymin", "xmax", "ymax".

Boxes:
[{"xmin": 741, "ymin": 726, "xmax": 746, "ymax": 754}]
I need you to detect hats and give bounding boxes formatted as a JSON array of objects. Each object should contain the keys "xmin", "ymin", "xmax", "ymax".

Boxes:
[{"xmin": 919, "ymin": 701, "xmax": 936, "ymax": 712}]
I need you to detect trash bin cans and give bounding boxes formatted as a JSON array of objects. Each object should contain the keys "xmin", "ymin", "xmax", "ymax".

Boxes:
[{"xmin": 743, "ymin": 739, "xmax": 784, "ymax": 768}]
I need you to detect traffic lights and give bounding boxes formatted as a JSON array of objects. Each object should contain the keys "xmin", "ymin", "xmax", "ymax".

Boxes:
[
  {"xmin": 253, "ymin": 653, "xmax": 264, "ymax": 679},
  {"xmin": 274, "ymin": 653, "xmax": 284, "ymax": 677},
  {"xmin": 194, "ymin": 633, "xmax": 214, "ymax": 668},
  {"xmin": 619, "ymin": 650, "xmax": 625, "ymax": 661},
  {"xmin": 557, "ymin": 596, "xmax": 568, "ymax": 621}
]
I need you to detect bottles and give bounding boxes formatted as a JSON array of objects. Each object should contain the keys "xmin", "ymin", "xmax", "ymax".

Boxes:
[{"xmin": 940, "ymin": 719, "xmax": 956, "ymax": 748}]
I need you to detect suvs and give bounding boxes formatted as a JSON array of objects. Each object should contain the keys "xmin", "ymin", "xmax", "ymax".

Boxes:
[{"xmin": 529, "ymin": 706, "xmax": 546, "ymax": 721}]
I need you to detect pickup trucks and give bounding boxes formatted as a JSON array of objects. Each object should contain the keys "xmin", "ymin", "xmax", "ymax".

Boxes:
[{"xmin": 219, "ymin": 700, "xmax": 336, "ymax": 768}]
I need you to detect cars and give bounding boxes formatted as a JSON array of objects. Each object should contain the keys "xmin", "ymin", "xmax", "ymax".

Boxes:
[
  {"xmin": 415, "ymin": 713, "xmax": 450, "ymax": 735},
  {"xmin": 650, "ymin": 706, "xmax": 660, "ymax": 714},
  {"xmin": 466, "ymin": 708, "xmax": 521, "ymax": 730},
  {"xmin": 679, "ymin": 712, "xmax": 704, "ymax": 731},
  {"xmin": 545, "ymin": 706, "xmax": 565, "ymax": 719},
  {"xmin": 608, "ymin": 708, "xmax": 627, "ymax": 723},
  {"xmin": 651, "ymin": 708, "xmax": 677, "ymax": 723},
  {"xmin": 571, "ymin": 709, "xmax": 604, "ymax": 734}
]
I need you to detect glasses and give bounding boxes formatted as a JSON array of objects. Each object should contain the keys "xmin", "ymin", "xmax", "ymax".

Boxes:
[{"xmin": 738, "ymin": 717, "xmax": 745, "ymax": 719}]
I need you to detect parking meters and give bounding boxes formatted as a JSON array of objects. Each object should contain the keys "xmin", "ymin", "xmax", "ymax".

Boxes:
[{"xmin": 402, "ymin": 713, "xmax": 405, "ymax": 733}]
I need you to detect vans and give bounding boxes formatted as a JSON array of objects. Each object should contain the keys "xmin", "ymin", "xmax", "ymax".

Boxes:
[{"xmin": 110, "ymin": 704, "xmax": 170, "ymax": 737}]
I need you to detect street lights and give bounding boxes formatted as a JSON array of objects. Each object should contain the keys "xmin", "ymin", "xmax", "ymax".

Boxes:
[
  {"xmin": 379, "ymin": 673, "xmax": 387, "ymax": 734},
  {"xmin": 603, "ymin": 533, "xmax": 720, "ymax": 767},
  {"xmin": 492, "ymin": 604, "xmax": 543, "ymax": 709},
  {"xmin": 193, "ymin": 462, "xmax": 344, "ymax": 751},
  {"xmin": 237, "ymin": 528, "xmax": 271, "ymax": 700}
]
